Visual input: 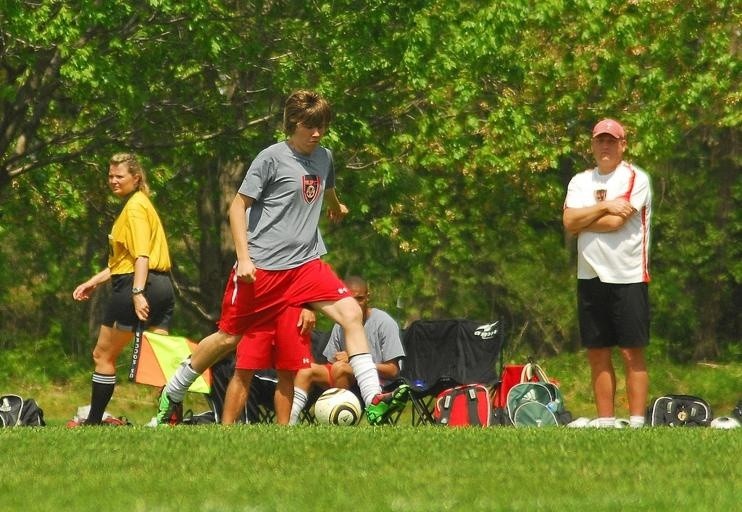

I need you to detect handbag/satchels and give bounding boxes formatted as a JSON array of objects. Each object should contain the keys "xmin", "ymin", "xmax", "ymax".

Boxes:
[
  {"xmin": 0, "ymin": 393, "xmax": 45, "ymax": 427},
  {"xmin": 646, "ymin": 394, "xmax": 712, "ymax": 429},
  {"xmin": 434, "ymin": 383, "xmax": 491, "ymax": 428},
  {"xmin": 505, "ymin": 364, "xmax": 574, "ymax": 431},
  {"xmin": 66, "ymin": 406, "xmax": 131, "ymax": 426},
  {"xmin": 490, "ymin": 363, "xmax": 559, "ymax": 426}
]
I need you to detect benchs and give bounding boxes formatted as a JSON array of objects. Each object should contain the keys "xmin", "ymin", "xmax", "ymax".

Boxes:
[{"xmin": 259, "ymin": 318, "xmax": 505, "ymax": 425}]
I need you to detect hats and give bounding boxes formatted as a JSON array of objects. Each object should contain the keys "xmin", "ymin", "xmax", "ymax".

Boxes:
[{"xmin": 592, "ymin": 119, "xmax": 624, "ymax": 139}]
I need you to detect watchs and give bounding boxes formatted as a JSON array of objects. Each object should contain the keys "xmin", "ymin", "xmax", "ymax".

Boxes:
[{"xmin": 132, "ymin": 287, "xmax": 145, "ymax": 295}]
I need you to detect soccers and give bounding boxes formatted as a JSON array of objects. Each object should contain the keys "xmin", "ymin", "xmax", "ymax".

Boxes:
[{"xmin": 314, "ymin": 388, "xmax": 362, "ymax": 424}]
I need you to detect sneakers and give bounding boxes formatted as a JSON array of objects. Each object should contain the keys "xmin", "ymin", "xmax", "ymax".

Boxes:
[
  {"xmin": 367, "ymin": 382, "xmax": 410, "ymax": 426},
  {"xmin": 141, "ymin": 416, "xmax": 167, "ymax": 427}
]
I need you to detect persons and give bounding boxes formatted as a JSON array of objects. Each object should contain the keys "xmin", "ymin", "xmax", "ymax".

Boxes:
[
  {"xmin": 289, "ymin": 277, "xmax": 405, "ymax": 424},
  {"xmin": 563, "ymin": 120, "xmax": 651, "ymax": 429},
  {"xmin": 67, "ymin": 152, "xmax": 175, "ymax": 428},
  {"xmin": 223, "ymin": 301, "xmax": 315, "ymax": 424},
  {"xmin": 158, "ymin": 90, "xmax": 409, "ymax": 424}
]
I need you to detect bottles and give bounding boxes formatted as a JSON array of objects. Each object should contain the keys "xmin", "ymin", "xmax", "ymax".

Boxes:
[
  {"xmin": 548, "ymin": 399, "xmax": 561, "ymax": 412},
  {"xmin": 413, "ymin": 379, "xmax": 427, "ymax": 391}
]
